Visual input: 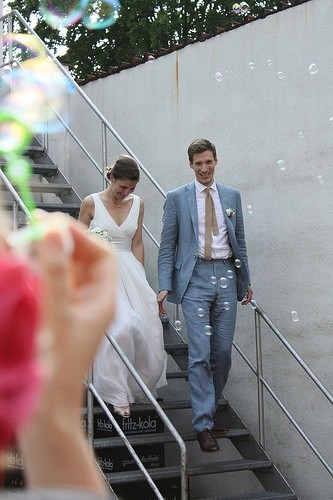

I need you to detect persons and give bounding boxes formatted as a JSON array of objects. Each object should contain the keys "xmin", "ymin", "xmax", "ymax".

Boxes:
[
  {"xmin": 0, "ymin": 207, "xmax": 119, "ymax": 500},
  {"xmin": 77, "ymin": 156, "xmax": 168, "ymax": 418},
  {"xmin": 156, "ymin": 139, "xmax": 253, "ymax": 452}
]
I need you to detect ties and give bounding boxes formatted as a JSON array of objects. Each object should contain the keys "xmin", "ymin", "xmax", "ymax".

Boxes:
[{"xmin": 204, "ymin": 188, "xmax": 220, "ymax": 259}]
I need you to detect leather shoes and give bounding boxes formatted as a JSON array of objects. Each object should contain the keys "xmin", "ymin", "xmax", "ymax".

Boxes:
[
  {"xmin": 213, "ymin": 420, "xmax": 224, "ymax": 436},
  {"xmin": 197, "ymin": 430, "xmax": 220, "ymax": 452}
]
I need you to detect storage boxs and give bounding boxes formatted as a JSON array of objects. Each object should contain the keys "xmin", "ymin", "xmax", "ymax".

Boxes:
[{"xmin": 6, "ymin": 390, "xmax": 190, "ymax": 500}]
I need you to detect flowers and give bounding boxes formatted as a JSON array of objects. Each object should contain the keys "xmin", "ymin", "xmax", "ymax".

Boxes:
[
  {"xmin": 88, "ymin": 227, "xmax": 113, "ymax": 250},
  {"xmin": 225, "ymin": 206, "xmax": 236, "ymax": 218}
]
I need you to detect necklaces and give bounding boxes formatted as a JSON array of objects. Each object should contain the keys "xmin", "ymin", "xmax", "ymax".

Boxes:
[{"xmin": 110, "ymin": 186, "xmax": 127, "ymax": 207}]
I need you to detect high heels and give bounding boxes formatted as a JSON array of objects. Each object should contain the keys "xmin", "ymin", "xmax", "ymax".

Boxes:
[{"xmin": 107, "ymin": 401, "xmax": 131, "ymax": 418}]
